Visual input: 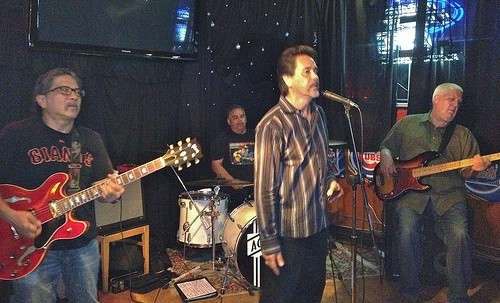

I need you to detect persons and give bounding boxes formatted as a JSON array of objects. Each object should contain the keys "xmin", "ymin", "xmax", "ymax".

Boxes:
[
  {"xmin": 254, "ymin": 45, "xmax": 344, "ymax": 303},
  {"xmin": 0, "ymin": 68, "xmax": 125, "ymax": 303},
  {"xmin": 380, "ymin": 83, "xmax": 491, "ymax": 303},
  {"xmin": 209, "ymin": 104, "xmax": 258, "ymax": 206}
]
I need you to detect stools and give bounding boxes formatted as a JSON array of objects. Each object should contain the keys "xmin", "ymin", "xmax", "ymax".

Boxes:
[{"xmin": 96, "ymin": 222, "xmax": 150, "ymax": 294}]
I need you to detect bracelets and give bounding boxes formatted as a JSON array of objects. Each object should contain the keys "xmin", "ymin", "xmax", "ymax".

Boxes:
[{"xmin": 112, "ymin": 196, "xmax": 121, "ymax": 204}]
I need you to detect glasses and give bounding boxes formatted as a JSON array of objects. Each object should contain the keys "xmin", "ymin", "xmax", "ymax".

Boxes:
[{"xmin": 42, "ymin": 86, "xmax": 86, "ymax": 97}]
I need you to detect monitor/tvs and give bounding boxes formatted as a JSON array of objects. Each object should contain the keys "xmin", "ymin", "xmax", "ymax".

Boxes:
[{"xmin": 27, "ymin": 0, "xmax": 201, "ymax": 61}]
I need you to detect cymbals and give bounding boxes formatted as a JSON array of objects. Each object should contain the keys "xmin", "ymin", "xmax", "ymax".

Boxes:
[{"xmin": 185, "ymin": 177, "xmax": 254, "ymax": 187}]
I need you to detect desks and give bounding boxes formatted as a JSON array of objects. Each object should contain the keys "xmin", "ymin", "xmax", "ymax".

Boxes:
[{"xmin": 325, "ymin": 174, "xmax": 500, "ymax": 282}]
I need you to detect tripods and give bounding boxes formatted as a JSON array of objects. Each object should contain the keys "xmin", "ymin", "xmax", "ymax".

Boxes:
[{"xmin": 162, "ymin": 187, "xmax": 254, "ymax": 296}]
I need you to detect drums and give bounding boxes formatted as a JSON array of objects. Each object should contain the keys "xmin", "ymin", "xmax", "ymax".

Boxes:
[
  {"xmin": 176, "ymin": 190, "xmax": 230, "ymax": 249},
  {"xmin": 327, "ymin": 139, "xmax": 348, "ymax": 179},
  {"xmin": 222, "ymin": 199, "xmax": 272, "ymax": 290}
]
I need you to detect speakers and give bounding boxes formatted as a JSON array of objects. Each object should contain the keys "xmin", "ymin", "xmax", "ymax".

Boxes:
[{"xmin": 92, "ymin": 178, "xmax": 147, "ymax": 234}]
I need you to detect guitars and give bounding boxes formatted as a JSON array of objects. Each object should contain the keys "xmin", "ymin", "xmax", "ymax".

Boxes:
[
  {"xmin": 0, "ymin": 137, "xmax": 202, "ymax": 280},
  {"xmin": 373, "ymin": 151, "xmax": 500, "ymax": 202}
]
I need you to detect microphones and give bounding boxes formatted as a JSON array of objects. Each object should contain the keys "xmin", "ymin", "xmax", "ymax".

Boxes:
[{"xmin": 321, "ymin": 90, "xmax": 359, "ymax": 108}]
